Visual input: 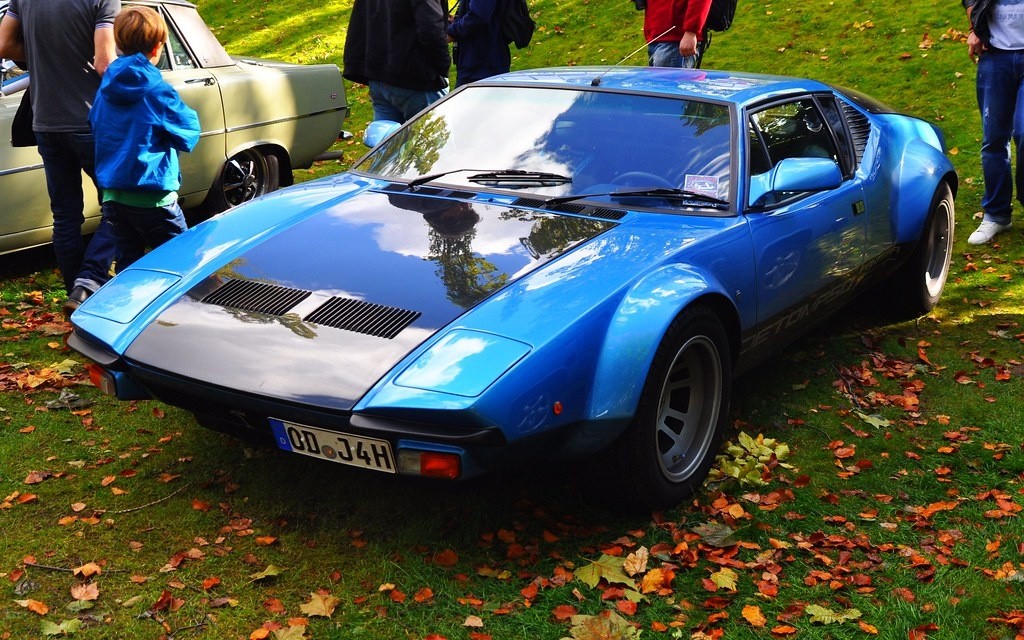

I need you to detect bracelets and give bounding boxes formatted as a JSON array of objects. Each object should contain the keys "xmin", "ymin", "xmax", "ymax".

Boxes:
[{"xmin": 968, "ymin": 25, "xmax": 974, "ymax": 34}]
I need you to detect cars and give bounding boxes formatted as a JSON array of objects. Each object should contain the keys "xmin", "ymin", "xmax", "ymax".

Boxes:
[{"xmin": 1, "ymin": 0, "xmax": 352, "ymax": 256}]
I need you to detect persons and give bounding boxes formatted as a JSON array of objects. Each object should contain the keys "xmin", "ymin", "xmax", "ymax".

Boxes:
[
  {"xmin": 0, "ymin": 0, "xmax": 120, "ymax": 316},
  {"xmin": 448, "ymin": 0, "xmax": 535, "ymax": 90},
  {"xmin": 88, "ymin": 6, "xmax": 201, "ymax": 275},
  {"xmin": 341, "ymin": 0, "xmax": 450, "ymax": 124},
  {"xmin": 961, "ymin": 0, "xmax": 1024, "ymax": 244},
  {"xmin": 644, "ymin": 0, "xmax": 713, "ymax": 69}
]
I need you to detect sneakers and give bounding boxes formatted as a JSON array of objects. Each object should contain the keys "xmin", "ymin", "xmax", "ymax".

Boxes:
[{"xmin": 968, "ymin": 220, "xmax": 1013, "ymax": 245}]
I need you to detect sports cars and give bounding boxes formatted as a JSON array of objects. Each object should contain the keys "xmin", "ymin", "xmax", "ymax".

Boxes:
[{"xmin": 66, "ymin": 64, "xmax": 958, "ymax": 510}]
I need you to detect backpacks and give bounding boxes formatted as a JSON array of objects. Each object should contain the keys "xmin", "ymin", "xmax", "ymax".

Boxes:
[{"xmin": 709, "ymin": 0, "xmax": 738, "ymax": 34}]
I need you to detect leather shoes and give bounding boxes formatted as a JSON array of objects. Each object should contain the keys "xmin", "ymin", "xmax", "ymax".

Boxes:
[{"xmin": 63, "ymin": 286, "xmax": 88, "ymax": 312}]
